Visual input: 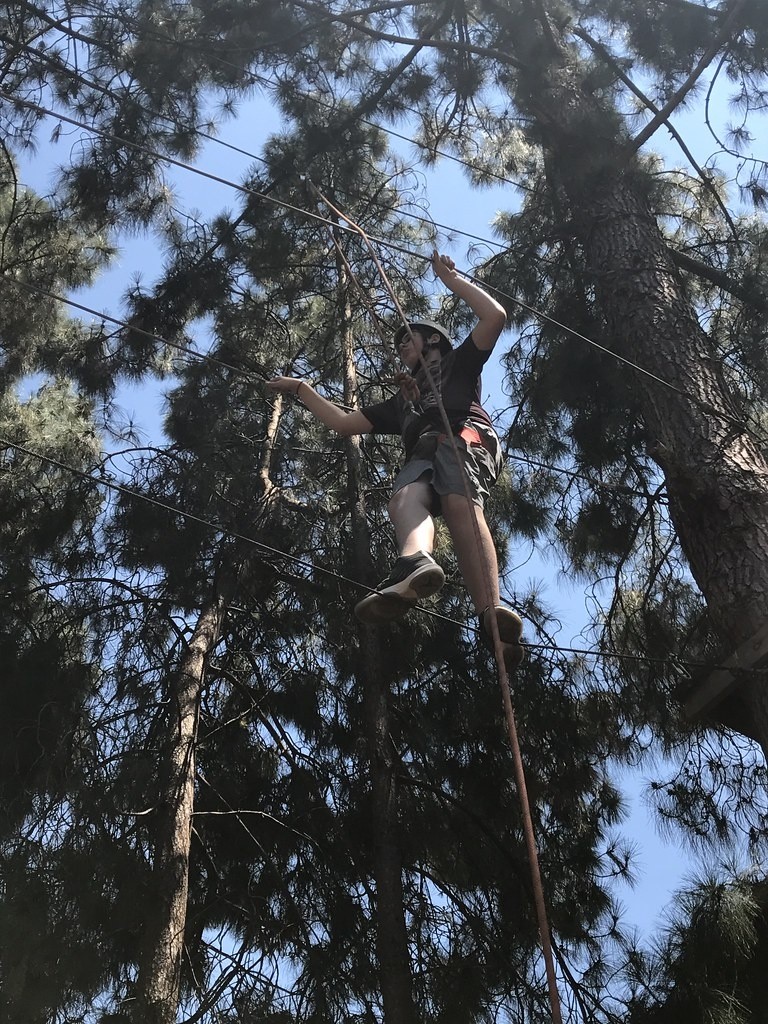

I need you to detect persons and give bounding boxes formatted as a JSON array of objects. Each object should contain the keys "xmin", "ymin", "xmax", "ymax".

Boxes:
[{"xmin": 266, "ymin": 249, "xmax": 524, "ymax": 672}]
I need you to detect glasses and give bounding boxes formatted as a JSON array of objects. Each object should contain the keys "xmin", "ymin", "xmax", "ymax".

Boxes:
[{"xmin": 402, "ymin": 330, "xmax": 420, "ymax": 343}]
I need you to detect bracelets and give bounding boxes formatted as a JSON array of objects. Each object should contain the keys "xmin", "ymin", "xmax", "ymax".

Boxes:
[{"xmin": 296, "ymin": 381, "xmax": 304, "ymax": 395}]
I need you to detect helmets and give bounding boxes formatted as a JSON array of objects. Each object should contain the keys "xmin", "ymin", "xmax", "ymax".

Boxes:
[{"xmin": 394, "ymin": 321, "xmax": 453, "ymax": 356}]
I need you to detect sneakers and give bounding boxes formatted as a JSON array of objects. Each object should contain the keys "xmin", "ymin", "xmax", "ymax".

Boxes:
[
  {"xmin": 354, "ymin": 552, "xmax": 445, "ymax": 625},
  {"xmin": 480, "ymin": 606, "xmax": 524, "ymax": 659}
]
